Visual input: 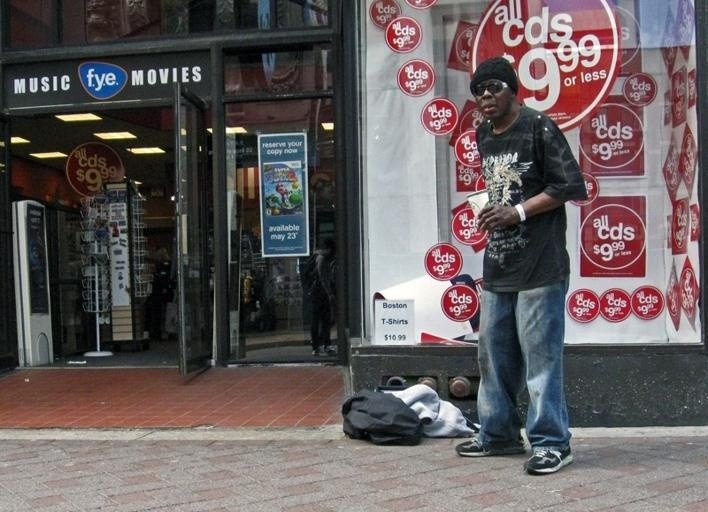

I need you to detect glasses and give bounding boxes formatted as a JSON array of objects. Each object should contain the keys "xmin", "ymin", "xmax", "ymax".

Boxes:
[{"xmin": 468, "ymin": 79, "xmax": 509, "ymax": 98}]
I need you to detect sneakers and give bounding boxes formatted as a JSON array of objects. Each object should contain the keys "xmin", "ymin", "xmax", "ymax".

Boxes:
[
  {"xmin": 525, "ymin": 440, "xmax": 576, "ymax": 476},
  {"xmin": 454, "ymin": 431, "xmax": 526, "ymax": 458},
  {"xmin": 313, "ymin": 344, "xmax": 338, "ymax": 356}
]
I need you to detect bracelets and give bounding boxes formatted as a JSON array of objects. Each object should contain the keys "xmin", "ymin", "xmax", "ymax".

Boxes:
[{"xmin": 516, "ymin": 203, "xmax": 526, "ymax": 222}]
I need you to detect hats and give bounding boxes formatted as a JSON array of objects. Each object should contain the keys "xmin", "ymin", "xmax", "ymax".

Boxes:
[{"xmin": 467, "ymin": 55, "xmax": 520, "ymax": 96}]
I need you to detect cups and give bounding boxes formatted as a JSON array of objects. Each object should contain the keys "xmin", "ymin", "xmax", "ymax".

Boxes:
[{"xmin": 467, "ymin": 188, "xmax": 492, "ymax": 220}]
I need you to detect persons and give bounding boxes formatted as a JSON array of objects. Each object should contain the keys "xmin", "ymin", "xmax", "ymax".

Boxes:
[
  {"xmin": 455, "ymin": 57, "xmax": 589, "ymax": 475},
  {"xmin": 305, "ymin": 238, "xmax": 338, "ymax": 357}
]
